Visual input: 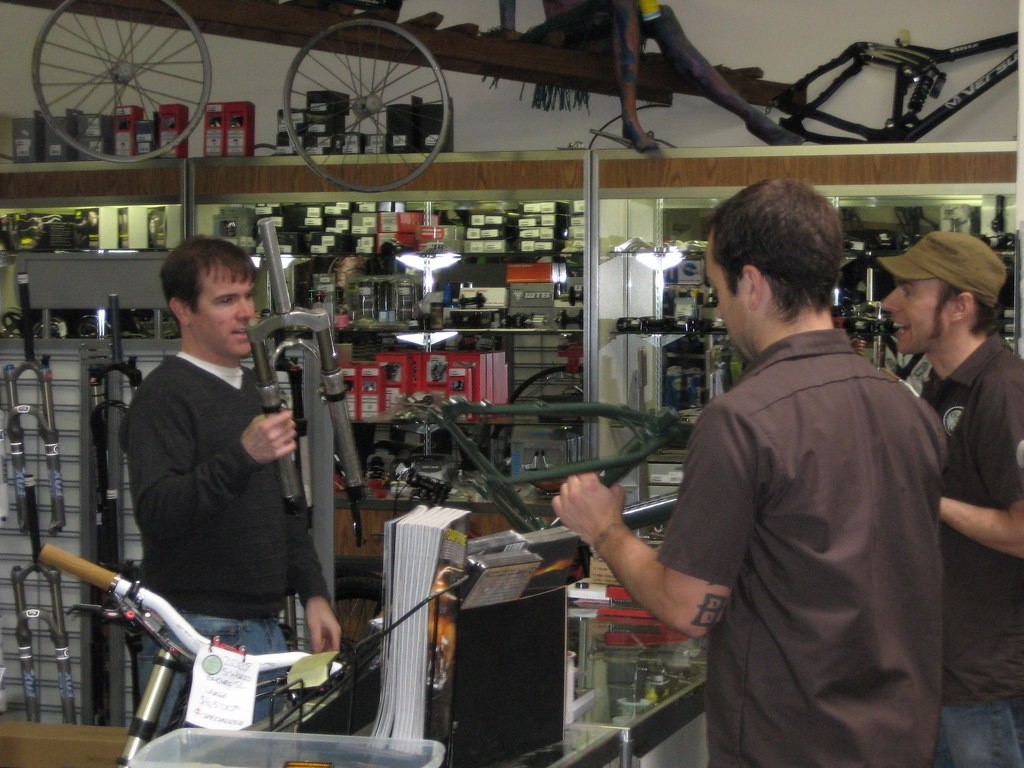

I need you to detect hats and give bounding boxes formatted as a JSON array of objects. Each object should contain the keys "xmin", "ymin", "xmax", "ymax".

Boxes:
[{"xmin": 875, "ymin": 232, "xmax": 1008, "ymax": 307}]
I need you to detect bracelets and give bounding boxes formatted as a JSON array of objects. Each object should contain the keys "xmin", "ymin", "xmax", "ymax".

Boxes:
[{"xmin": 589, "ymin": 523, "xmax": 626, "ymax": 560}]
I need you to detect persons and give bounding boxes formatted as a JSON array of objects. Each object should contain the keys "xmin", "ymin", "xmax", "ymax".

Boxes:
[
  {"xmin": 499, "ymin": 0, "xmax": 806, "ymax": 153},
  {"xmin": 879, "ymin": 230, "xmax": 1024, "ymax": 768},
  {"xmin": 117, "ymin": 234, "xmax": 342, "ymax": 738},
  {"xmin": 549, "ymin": 177, "xmax": 950, "ymax": 768}
]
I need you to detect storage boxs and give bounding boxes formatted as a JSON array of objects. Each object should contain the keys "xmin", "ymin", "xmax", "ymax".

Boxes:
[
  {"xmin": 251, "ymin": 202, "xmax": 583, "ymax": 420},
  {"xmin": 125, "ymin": 726, "xmax": 445, "ymax": 768},
  {"xmin": 11, "ymin": 91, "xmax": 453, "ymax": 164}
]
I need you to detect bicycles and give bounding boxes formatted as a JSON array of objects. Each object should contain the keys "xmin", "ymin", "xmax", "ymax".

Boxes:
[{"xmin": 35, "ymin": 542, "xmax": 449, "ymax": 768}]
